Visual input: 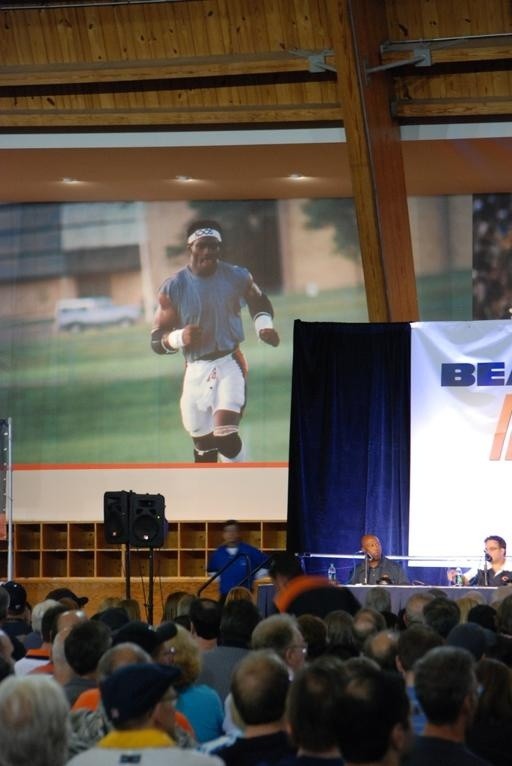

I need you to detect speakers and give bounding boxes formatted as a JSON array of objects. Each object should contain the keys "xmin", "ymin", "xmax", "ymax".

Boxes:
[{"xmin": 104, "ymin": 491, "xmax": 165, "ymax": 548}]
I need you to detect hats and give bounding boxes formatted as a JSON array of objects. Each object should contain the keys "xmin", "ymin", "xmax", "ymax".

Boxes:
[
  {"xmin": 1, "ymin": 582, "xmax": 25, "ymax": 611},
  {"xmin": 112, "ymin": 622, "xmax": 177, "ymax": 654},
  {"xmin": 99, "ymin": 664, "xmax": 181, "ymax": 722},
  {"xmin": 448, "ymin": 623, "xmax": 496, "ymax": 660},
  {"xmin": 46, "ymin": 588, "xmax": 88, "ymax": 609}
]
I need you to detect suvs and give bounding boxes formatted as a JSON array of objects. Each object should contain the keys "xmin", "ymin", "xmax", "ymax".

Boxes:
[{"xmin": 57, "ymin": 297, "xmax": 139, "ymax": 335}]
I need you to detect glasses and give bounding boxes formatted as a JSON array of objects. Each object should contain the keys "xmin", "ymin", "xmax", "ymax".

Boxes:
[
  {"xmin": 292, "ymin": 642, "xmax": 308, "ymax": 655},
  {"xmin": 164, "ymin": 646, "xmax": 175, "ymax": 654}
]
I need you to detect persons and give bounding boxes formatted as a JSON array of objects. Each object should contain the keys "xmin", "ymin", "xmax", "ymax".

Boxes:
[
  {"xmin": 0, "ymin": 553, "xmax": 512, "ymax": 766},
  {"xmin": 206, "ymin": 520, "xmax": 273, "ymax": 604},
  {"xmin": 151, "ymin": 220, "xmax": 279, "ymax": 463},
  {"xmin": 349, "ymin": 534, "xmax": 410, "ymax": 583},
  {"xmin": 447, "ymin": 535, "xmax": 512, "ymax": 585}
]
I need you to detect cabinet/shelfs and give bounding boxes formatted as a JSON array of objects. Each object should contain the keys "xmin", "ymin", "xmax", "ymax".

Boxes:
[{"xmin": 0, "ymin": 521, "xmax": 287, "ymax": 628}]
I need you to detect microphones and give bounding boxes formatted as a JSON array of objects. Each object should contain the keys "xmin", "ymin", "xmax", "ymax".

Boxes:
[
  {"xmin": 355, "ymin": 551, "xmax": 364, "ymax": 554},
  {"xmin": 484, "ymin": 551, "xmax": 493, "ymax": 562}
]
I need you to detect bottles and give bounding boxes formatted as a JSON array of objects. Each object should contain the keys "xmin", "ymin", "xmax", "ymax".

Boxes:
[
  {"xmin": 454, "ymin": 566, "xmax": 463, "ymax": 588},
  {"xmin": 328, "ymin": 562, "xmax": 336, "ymax": 586}
]
